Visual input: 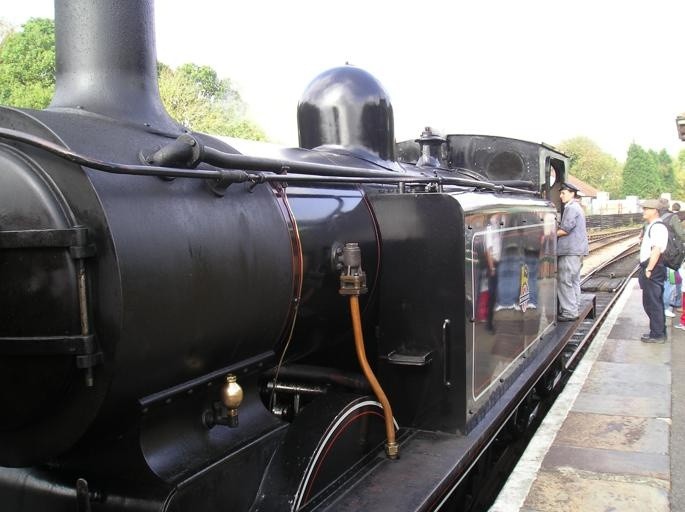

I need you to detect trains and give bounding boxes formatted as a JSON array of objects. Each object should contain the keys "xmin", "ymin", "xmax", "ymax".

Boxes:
[{"xmin": 1, "ymin": 0, "xmax": 596, "ymax": 511}]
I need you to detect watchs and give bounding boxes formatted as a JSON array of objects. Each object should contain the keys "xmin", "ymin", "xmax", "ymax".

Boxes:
[{"xmin": 645, "ymin": 267, "xmax": 654, "ymax": 272}]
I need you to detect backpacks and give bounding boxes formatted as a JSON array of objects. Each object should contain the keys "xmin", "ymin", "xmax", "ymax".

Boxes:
[{"xmin": 639, "ymin": 221, "xmax": 685, "ymax": 271}]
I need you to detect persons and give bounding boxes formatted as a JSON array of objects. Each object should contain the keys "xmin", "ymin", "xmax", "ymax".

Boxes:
[
  {"xmin": 556, "ymin": 181, "xmax": 590, "ymax": 321},
  {"xmin": 638, "ymin": 199, "xmax": 671, "ymax": 344},
  {"xmin": 461, "ymin": 210, "xmax": 557, "ymax": 334},
  {"xmin": 659, "ymin": 198, "xmax": 685, "ymax": 331}
]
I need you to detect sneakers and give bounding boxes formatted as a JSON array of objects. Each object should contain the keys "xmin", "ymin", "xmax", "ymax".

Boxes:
[
  {"xmin": 673, "ymin": 324, "xmax": 685, "ymax": 331},
  {"xmin": 664, "ymin": 309, "xmax": 676, "ymax": 318},
  {"xmin": 642, "ymin": 333, "xmax": 667, "ymax": 340},
  {"xmin": 557, "ymin": 315, "xmax": 579, "ymax": 322},
  {"xmin": 640, "ymin": 337, "xmax": 665, "ymax": 344}
]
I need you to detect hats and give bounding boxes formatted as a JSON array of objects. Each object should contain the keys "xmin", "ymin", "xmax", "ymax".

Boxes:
[
  {"xmin": 661, "ymin": 199, "xmax": 669, "ymax": 208},
  {"xmin": 639, "ymin": 199, "xmax": 660, "ymax": 210},
  {"xmin": 558, "ymin": 182, "xmax": 580, "ymax": 193}
]
